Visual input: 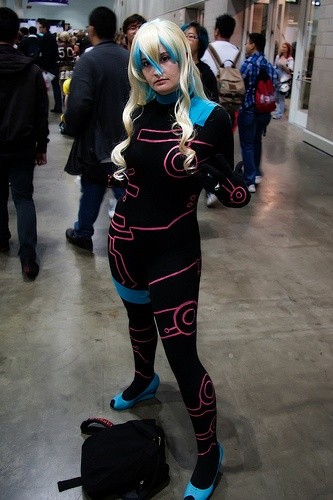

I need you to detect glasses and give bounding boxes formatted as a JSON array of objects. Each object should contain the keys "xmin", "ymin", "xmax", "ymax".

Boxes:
[{"xmin": 186, "ymin": 35, "xmax": 198, "ymax": 40}]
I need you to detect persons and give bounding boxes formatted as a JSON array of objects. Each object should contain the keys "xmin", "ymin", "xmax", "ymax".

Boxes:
[
  {"xmin": 0, "ymin": 7, "xmax": 50, "ymax": 280},
  {"xmin": 106, "ymin": 19, "xmax": 251, "ymax": 500},
  {"xmin": 181, "ymin": 22, "xmax": 219, "ymax": 104},
  {"xmin": 201, "ymin": 14, "xmax": 244, "ymax": 206},
  {"xmin": 272, "ymin": 43, "xmax": 294, "ymax": 119},
  {"xmin": 239, "ymin": 33, "xmax": 281, "ymax": 192},
  {"xmin": 15, "ymin": 18, "xmax": 92, "ymax": 114},
  {"xmin": 62, "ymin": 6, "xmax": 131, "ymax": 251},
  {"xmin": 116, "ymin": 14, "xmax": 146, "ymax": 50}
]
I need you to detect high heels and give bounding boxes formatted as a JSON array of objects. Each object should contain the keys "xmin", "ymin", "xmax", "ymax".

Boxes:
[
  {"xmin": 110, "ymin": 373, "xmax": 160, "ymax": 410},
  {"xmin": 183, "ymin": 441, "xmax": 223, "ymax": 500}
]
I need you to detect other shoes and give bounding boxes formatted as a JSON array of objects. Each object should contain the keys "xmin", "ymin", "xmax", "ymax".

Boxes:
[
  {"xmin": 206, "ymin": 193, "xmax": 218, "ymax": 207},
  {"xmin": 65, "ymin": 228, "xmax": 93, "ymax": 254},
  {"xmin": 51, "ymin": 109, "xmax": 62, "ymax": 113},
  {"xmin": 255, "ymin": 176, "xmax": 262, "ymax": 184},
  {"xmin": 248, "ymin": 184, "xmax": 256, "ymax": 193},
  {"xmin": 23, "ymin": 262, "xmax": 39, "ymax": 281},
  {"xmin": 273, "ymin": 116, "xmax": 278, "ymax": 119}
]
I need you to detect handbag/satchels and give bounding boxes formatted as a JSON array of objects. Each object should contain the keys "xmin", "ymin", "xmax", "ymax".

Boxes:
[{"xmin": 56, "ymin": 418, "xmax": 170, "ymax": 500}]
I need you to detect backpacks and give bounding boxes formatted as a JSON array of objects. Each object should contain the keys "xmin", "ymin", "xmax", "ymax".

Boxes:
[
  {"xmin": 206, "ymin": 42, "xmax": 245, "ymax": 112},
  {"xmin": 246, "ymin": 61, "xmax": 276, "ymax": 113}
]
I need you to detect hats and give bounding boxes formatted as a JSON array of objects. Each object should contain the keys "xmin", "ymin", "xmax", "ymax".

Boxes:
[{"xmin": 60, "ymin": 32, "xmax": 69, "ymax": 41}]
